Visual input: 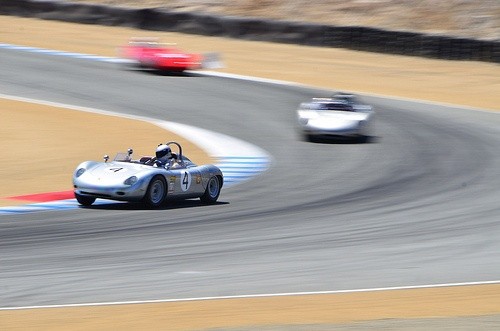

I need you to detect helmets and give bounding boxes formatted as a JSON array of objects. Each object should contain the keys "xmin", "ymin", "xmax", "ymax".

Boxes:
[{"xmin": 155, "ymin": 144, "xmax": 172, "ymax": 164}]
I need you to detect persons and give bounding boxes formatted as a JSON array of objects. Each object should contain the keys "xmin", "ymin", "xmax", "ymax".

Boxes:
[{"xmin": 152, "ymin": 145, "xmax": 178, "ymax": 169}]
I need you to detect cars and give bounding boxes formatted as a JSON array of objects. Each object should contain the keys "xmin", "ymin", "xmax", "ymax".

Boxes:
[{"xmin": 73, "ymin": 142, "xmax": 224, "ymax": 209}]
